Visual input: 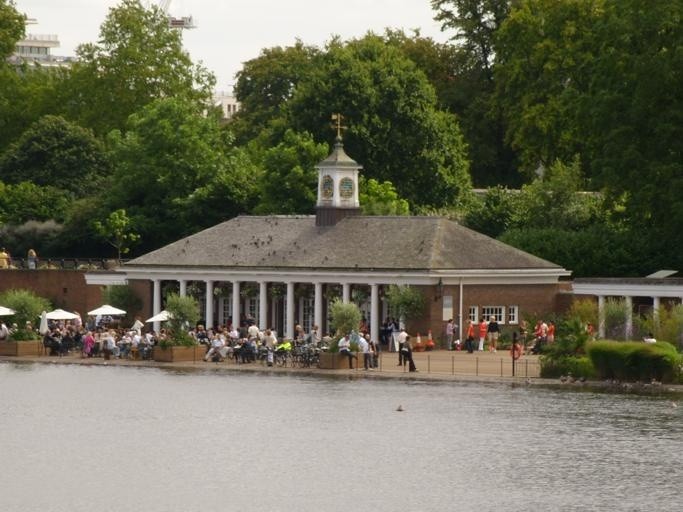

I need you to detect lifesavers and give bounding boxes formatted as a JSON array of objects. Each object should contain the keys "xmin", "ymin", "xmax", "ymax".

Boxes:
[{"xmin": 510, "ymin": 342, "xmax": 521, "ymax": 360}]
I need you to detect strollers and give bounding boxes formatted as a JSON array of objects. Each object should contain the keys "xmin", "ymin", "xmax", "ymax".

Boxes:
[{"xmin": 526, "ymin": 334, "xmax": 548, "ymax": 356}]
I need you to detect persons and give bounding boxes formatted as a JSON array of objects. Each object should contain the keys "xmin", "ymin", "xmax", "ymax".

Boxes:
[
  {"xmin": 446, "ymin": 314, "xmax": 597, "ymax": 355},
  {"xmin": 0, "ymin": 246, "xmax": 41, "ymax": 270},
  {"xmin": 188, "ymin": 315, "xmax": 422, "ymax": 373},
  {"xmin": 643, "ymin": 331, "xmax": 657, "ymax": 342},
  {"xmin": 0, "ymin": 316, "xmax": 176, "ymax": 361}
]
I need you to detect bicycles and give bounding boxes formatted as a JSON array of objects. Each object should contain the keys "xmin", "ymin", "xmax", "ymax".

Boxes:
[{"xmin": 223, "ymin": 336, "xmax": 333, "ymax": 369}]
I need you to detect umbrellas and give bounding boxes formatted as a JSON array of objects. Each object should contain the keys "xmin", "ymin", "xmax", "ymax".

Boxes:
[
  {"xmin": 0, "ymin": 306, "xmax": 17, "ymax": 316},
  {"xmin": 39, "ymin": 308, "xmax": 82, "ymax": 321},
  {"xmin": 86, "ymin": 303, "xmax": 128, "ymax": 316},
  {"xmin": 145, "ymin": 308, "xmax": 184, "ymax": 323}
]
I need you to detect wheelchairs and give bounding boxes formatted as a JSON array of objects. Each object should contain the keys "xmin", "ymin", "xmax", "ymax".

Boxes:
[{"xmin": 43, "ymin": 331, "xmax": 68, "ymax": 357}]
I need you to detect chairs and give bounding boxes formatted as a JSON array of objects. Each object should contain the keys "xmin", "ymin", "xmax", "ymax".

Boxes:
[
  {"xmin": 129, "ymin": 338, "xmax": 145, "ymax": 361},
  {"xmin": 238, "ymin": 345, "xmax": 257, "ymax": 364}
]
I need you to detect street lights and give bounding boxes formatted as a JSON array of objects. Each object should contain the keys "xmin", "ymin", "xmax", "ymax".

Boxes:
[
  {"xmin": 413, "ymin": 332, "xmax": 423, "ymax": 351},
  {"xmin": 424, "ymin": 329, "xmax": 434, "ymax": 347}
]
